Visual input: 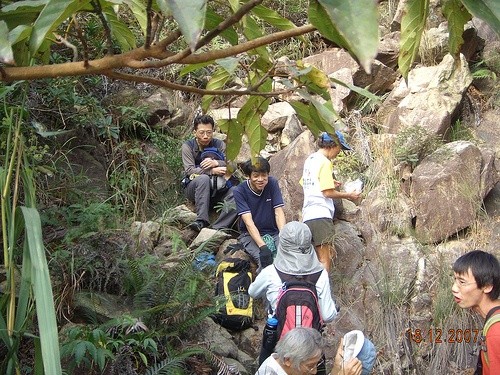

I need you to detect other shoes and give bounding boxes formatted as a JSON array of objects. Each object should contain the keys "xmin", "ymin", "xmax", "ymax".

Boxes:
[{"xmin": 188, "ymin": 220, "xmax": 203, "ymax": 232}]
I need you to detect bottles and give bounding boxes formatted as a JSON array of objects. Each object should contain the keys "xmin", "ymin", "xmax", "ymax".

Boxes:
[{"xmin": 265, "ymin": 318, "xmax": 278, "ymax": 330}]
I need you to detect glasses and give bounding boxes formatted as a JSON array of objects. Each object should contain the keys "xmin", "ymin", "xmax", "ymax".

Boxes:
[
  {"xmin": 199, "ymin": 131, "xmax": 212, "ymax": 137},
  {"xmin": 451, "ymin": 275, "xmax": 476, "ymax": 288}
]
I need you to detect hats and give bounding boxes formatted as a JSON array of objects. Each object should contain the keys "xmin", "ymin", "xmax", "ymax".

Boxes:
[
  {"xmin": 321, "ymin": 129, "xmax": 350, "ymax": 150},
  {"xmin": 272, "ymin": 219, "xmax": 325, "ymax": 276},
  {"xmin": 341, "ymin": 328, "xmax": 377, "ymax": 375}
]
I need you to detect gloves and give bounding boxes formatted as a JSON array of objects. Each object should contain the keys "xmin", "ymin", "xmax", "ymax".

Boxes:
[
  {"xmin": 259, "ymin": 245, "xmax": 274, "ymax": 268},
  {"xmin": 222, "ymin": 242, "xmax": 244, "ymax": 256}
]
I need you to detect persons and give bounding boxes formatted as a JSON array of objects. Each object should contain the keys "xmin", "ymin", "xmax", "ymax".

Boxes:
[
  {"xmin": 181, "ymin": 114, "xmax": 239, "ymax": 233},
  {"xmin": 249, "ymin": 221, "xmax": 339, "ymax": 375},
  {"xmin": 299, "ymin": 131, "xmax": 360, "ymax": 273},
  {"xmin": 234, "ymin": 157, "xmax": 287, "ymax": 275},
  {"xmin": 452, "ymin": 250, "xmax": 500, "ymax": 375},
  {"xmin": 255, "ymin": 327, "xmax": 363, "ymax": 375}
]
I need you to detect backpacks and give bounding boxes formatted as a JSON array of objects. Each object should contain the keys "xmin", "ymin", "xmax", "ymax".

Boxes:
[
  {"xmin": 272, "ymin": 264, "xmax": 324, "ymax": 340},
  {"xmin": 192, "ymin": 146, "xmax": 232, "ymax": 202},
  {"xmin": 212, "ymin": 257, "xmax": 258, "ymax": 333}
]
